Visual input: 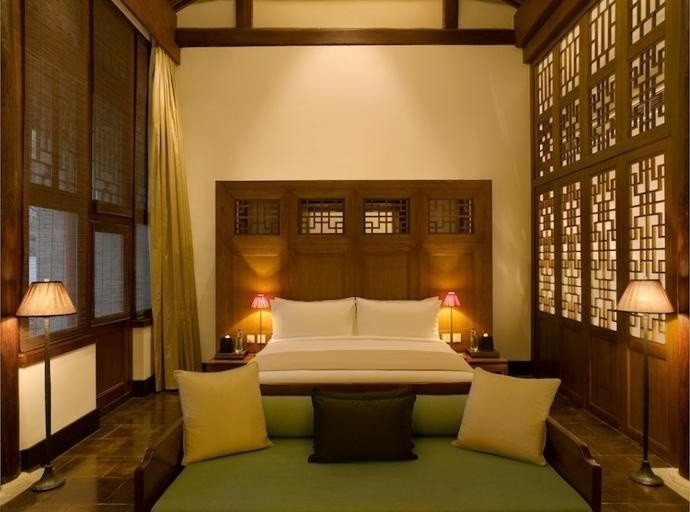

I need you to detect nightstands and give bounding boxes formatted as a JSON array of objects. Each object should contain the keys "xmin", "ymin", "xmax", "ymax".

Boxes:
[
  {"xmin": 464, "ymin": 354, "xmax": 509, "ymax": 377},
  {"xmin": 200, "ymin": 354, "xmax": 252, "ymax": 373}
]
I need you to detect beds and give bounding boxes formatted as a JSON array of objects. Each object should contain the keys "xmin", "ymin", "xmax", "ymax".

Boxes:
[{"xmin": 248, "ymin": 296, "xmax": 473, "ymax": 384}]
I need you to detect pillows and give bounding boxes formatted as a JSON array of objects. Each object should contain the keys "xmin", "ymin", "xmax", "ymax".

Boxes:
[
  {"xmin": 356, "ymin": 296, "xmax": 444, "ymax": 341},
  {"xmin": 306, "ymin": 385, "xmax": 424, "ymax": 464},
  {"xmin": 275, "ymin": 295, "xmax": 355, "ymax": 305},
  {"xmin": 451, "ymin": 366, "xmax": 565, "ymax": 469},
  {"xmin": 357, "ymin": 296, "xmax": 439, "ymax": 304},
  {"xmin": 172, "ymin": 361, "xmax": 273, "ymax": 468},
  {"xmin": 271, "ymin": 298, "xmax": 357, "ymax": 339}
]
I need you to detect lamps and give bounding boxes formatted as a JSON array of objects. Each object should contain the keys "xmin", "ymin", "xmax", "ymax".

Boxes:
[
  {"xmin": 250, "ymin": 294, "xmax": 270, "ymax": 355},
  {"xmin": 15, "ymin": 278, "xmax": 76, "ymax": 492},
  {"xmin": 618, "ymin": 277, "xmax": 675, "ymax": 488},
  {"xmin": 443, "ymin": 291, "xmax": 460, "ymax": 349}
]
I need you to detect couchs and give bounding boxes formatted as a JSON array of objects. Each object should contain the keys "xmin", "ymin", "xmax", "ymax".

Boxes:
[{"xmin": 132, "ymin": 382, "xmax": 603, "ymax": 511}]
